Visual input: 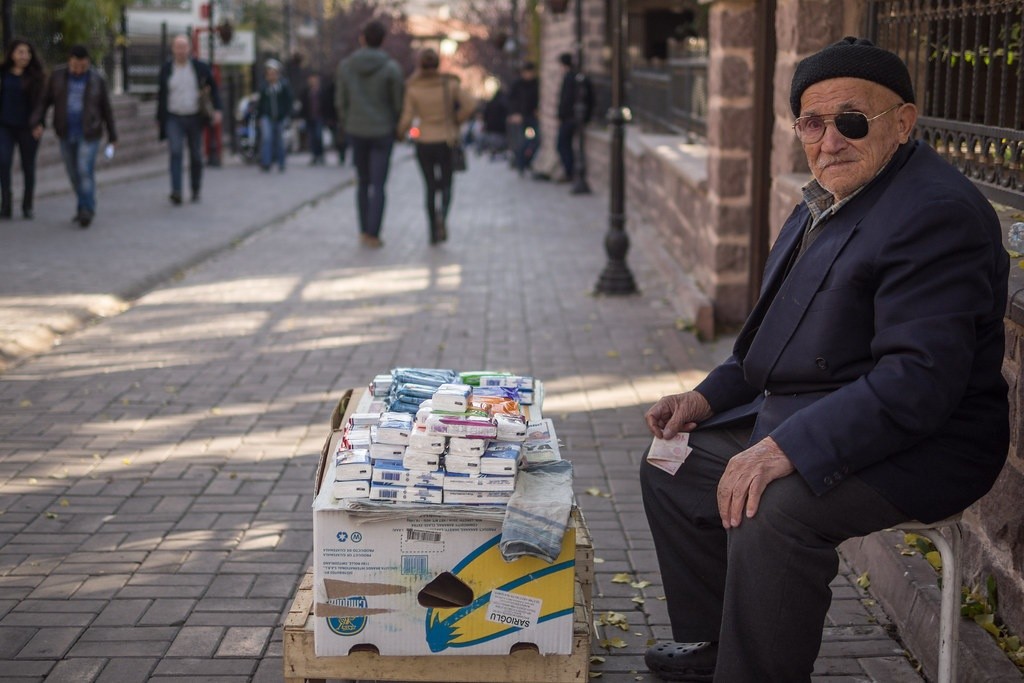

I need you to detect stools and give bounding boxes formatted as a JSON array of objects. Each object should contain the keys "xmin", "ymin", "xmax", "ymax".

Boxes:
[{"xmin": 886, "ymin": 516, "xmax": 963, "ymax": 683}]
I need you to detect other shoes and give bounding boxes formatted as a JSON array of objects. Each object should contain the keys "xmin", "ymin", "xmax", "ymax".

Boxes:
[
  {"xmin": 643, "ymin": 640, "xmax": 718, "ymax": 680},
  {"xmin": 77, "ymin": 206, "xmax": 93, "ymax": 230},
  {"xmin": 169, "ymin": 191, "xmax": 182, "ymax": 203},
  {"xmin": 192, "ymin": 188, "xmax": 201, "ymax": 200},
  {"xmin": 359, "ymin": 232, "xmax": 381, "ymax": 248},
  {"xmin": 433, "ymin": 220, "xmax": 447, "ymax": 239}
]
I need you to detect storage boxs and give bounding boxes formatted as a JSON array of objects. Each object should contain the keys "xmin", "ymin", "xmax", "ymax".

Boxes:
[{"xmin": 311, "ymin": 386, "xmax": 579, "ymax": 657}]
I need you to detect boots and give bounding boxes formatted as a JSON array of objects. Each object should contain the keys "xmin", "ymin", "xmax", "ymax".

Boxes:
[
  {"xmin": 20, "ymin": 195, "xmax": 36, "ymax": 220},
  {"xmin": 0, "ymin": 193, "xmax": 12, "ymax": 217}
]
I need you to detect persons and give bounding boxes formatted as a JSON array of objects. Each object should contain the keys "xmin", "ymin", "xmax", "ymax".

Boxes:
[
  {"xmin": 156, "ymin": 35, "xmax": 225, "ymax": 206},
  {"xmin": 32, "ymin": 46, "xmax": 118, "ymax": 230},
  {"xmin": 0, "ymin": 40, "xmax": 45, "ymax": 221},
  {"xmin": 256, "ymin": 58, "xmax": 543, "ymax": 179},
  {"xmin": 398, "ymin": 48, "xmax": 463, "ymax": 248},
  {"xmin": 640, "ymin": 36, "xmax": 1012, "ymax": 682},
  {"xmin": 552, "ymin": 51, "xmax": 590, "ymax": 187},
  {"xmin": 335, "ymin": 21, "xmax": 403, "ymax": 250}
]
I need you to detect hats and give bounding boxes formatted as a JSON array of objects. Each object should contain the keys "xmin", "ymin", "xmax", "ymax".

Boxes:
[
  {"xmin": 789, "ymin": 35, "xmax": 916, "ymax": 118},
  {"xmin": 264, "ymin": 58, "xmax": 282, "ymax": 72}
]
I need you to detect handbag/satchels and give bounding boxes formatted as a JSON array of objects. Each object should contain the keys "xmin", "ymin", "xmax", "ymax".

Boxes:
[
  {"xmin": 448, "ymin": 141, "xmax": 466, "ymax": 173},
  {"xmin": 198, "ymin": 95, "xmax": 217, "ymax": 130}
]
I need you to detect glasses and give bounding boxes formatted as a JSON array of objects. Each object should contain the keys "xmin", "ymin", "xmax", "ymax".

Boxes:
[{"xmin": 790, "ymin": 102, "xmax": 903, "ymax": 144}]
